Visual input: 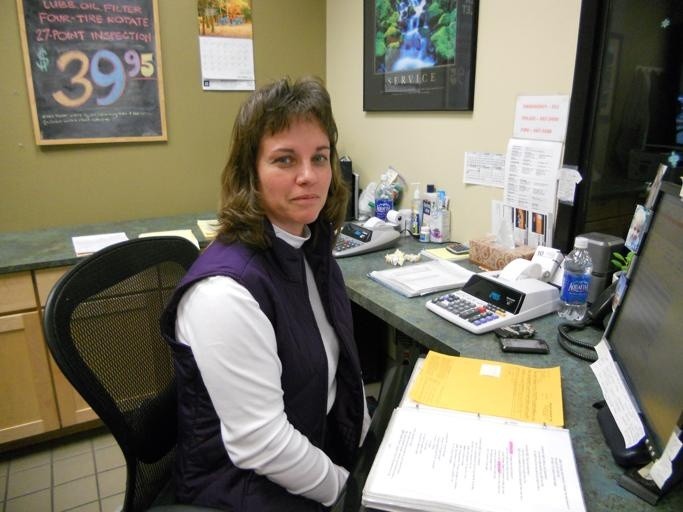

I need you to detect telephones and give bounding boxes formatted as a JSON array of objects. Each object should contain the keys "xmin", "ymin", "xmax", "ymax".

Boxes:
[{"xmin": 583, "ymin": 279, "xmax": 619, "ymax": 331}]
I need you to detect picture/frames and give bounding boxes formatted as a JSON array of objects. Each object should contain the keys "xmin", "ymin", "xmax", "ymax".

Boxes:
[{"xmin": 363, "ymin": 1, "xmax": 478, "ymax": 111}]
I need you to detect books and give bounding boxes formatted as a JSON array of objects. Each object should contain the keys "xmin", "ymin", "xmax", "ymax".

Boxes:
[
  {"xmin": 337, "ymin": 154, "xmax": 352, "ymax": 222},
  {"xmin": 359, "ymin": 350, "xmax": 589, "ymax": 512},
  {"xmin": 196, "ymin": 220, "xmax": 222, "ymax": 237},
  {"xmin": 350, "ymin": 170, "xmax": 359, "ymax": 221}
]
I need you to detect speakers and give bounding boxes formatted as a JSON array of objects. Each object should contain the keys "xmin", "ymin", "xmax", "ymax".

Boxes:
[{"xmin": 578, "ymin": 232, "xmax": 625, "ymax": 305}]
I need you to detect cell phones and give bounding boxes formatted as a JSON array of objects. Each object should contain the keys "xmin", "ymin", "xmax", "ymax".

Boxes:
[{"xmin": 499, "ymin": 338, "xmax": 550, "ymax": 353}]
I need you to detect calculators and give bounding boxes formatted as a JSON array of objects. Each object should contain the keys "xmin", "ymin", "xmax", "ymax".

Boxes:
[{"xmin": 446, "ymin": 243, "xmax": 470, "ymax": 254}]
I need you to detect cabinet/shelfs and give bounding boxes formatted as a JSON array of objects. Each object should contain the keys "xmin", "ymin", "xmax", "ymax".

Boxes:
[
  {"xmin": 584, "ymin": 195, "xmax": 646, "ymax": 241},
  {"xmin": 0, "ymin": 245, "xmax": 206, "ymax": 453}
]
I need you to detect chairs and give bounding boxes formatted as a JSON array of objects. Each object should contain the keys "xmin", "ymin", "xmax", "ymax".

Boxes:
[{"xmin": 42, "ymin": 237, "xmax": 201, "ymax": 511}]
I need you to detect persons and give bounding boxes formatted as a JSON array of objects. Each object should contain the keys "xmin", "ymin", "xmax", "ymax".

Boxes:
[
  {"xmin": 157, "ymin": 74, "xmax": 373, "ymax": 511},
  {"xmin": 625, "ymin": 206, "xmax": 646, "ymax": 251}
]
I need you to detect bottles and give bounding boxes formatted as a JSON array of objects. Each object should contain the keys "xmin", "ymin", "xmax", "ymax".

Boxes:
[
  {"xmin": 556, "ymin": 236, "xmax": 594, "ymax": 322},
  {"xmin": 374, "ymin": 174, "xmax": 395, "ymax": 221},
  {"xmin": 419, "ymin": 183, "xmax": 451, "ymax": 244}
]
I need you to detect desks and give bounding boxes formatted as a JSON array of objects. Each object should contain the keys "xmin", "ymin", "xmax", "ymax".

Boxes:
[{"xmin": 333, "ymin": 231, "xmax": 683, "ymax": 512}]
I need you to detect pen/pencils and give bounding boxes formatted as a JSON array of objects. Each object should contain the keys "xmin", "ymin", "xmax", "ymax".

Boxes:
[{"xmin": 439, "ymin": 190, "xmax": 449, "ymax": 210}]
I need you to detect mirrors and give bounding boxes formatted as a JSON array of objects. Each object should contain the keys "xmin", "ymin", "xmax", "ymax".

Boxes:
[{"xmin": 552, "ymin": 1, "xmax": 683, "ymax": 255}]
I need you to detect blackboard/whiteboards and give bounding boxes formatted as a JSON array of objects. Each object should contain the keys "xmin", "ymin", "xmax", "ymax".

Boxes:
[{"xmin": 16, "ymin": 0, "xmax": 168, "ymax": 146}]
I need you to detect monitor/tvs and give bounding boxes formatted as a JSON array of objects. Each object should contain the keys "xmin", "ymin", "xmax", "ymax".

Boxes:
[{"xmin": 590, "ymin": 182, "xmax": 683, "ymax": 490}]
